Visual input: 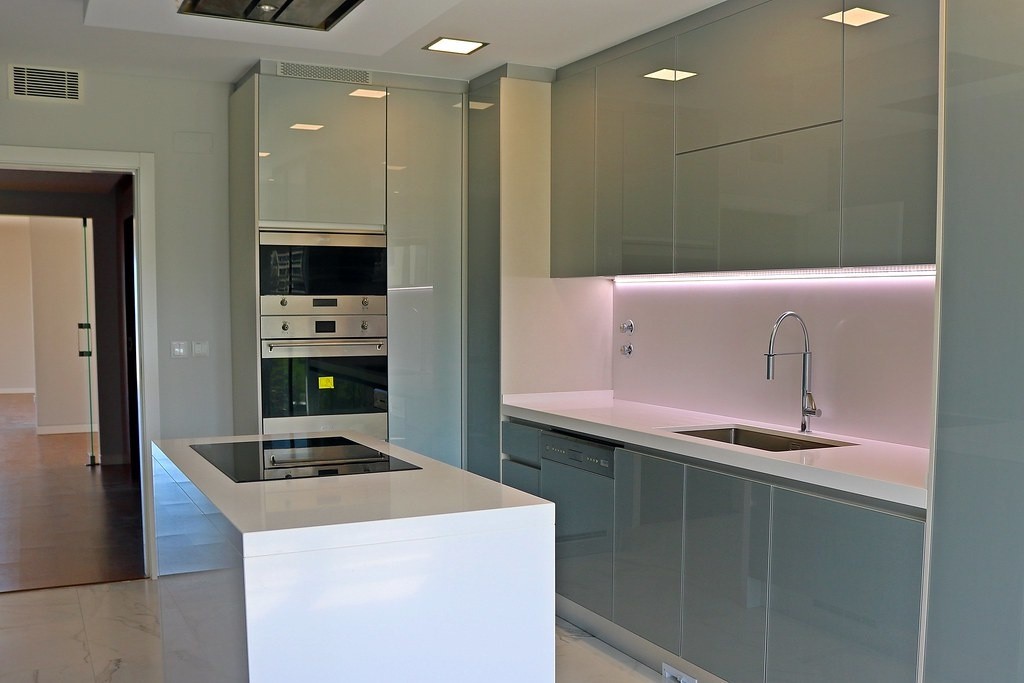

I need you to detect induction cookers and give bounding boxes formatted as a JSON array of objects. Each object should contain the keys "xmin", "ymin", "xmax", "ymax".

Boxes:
[{"xmin": 190, "ymin": 437, "xmax": 422, "ymax": 483}]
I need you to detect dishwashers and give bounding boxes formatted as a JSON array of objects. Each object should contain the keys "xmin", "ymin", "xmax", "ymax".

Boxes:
[{"xmin": 537, "ymin": 431, "xmax": 616, "ymax": 622}]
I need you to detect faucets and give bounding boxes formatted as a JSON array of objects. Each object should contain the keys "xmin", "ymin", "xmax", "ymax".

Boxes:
[{"xmin": 763, "ymin": 311, "xmax": 823, "ymax": 433}]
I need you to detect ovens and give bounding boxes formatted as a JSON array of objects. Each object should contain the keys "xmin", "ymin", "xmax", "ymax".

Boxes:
[{"xmin": 259, "ymin": 230, "xmax": 389, "ymax": 443}]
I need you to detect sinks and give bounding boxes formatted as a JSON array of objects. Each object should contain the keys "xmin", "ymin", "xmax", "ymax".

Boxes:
[{"xmin": 653, "ymin": 423, "xmax": 861, "ymax": 453}]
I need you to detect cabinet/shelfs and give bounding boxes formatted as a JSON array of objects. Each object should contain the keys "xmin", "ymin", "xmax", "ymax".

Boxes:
[
  {"xmin": 550, "ymin": 61, "xmax": 596, "ymax": 280},
  {"xmin": 227, "ymin": 76, "xmax": 388, "ymax": 231},
  {"xmin": 382, "ymin": 83, "xmax": 467, "ymax": 469},
  {"xmin": 768, "ymin": 484, "xmax": 926, "ymax": 683},
  {"xmin": 595, "ymin": 33, "xmax": 674, "ymax": 279},
  {"xmin": 611, "ymin": 446, "xmax": 770, "ymax": 683},
  {"xmin": 502, "ymin": 418, "xmax": 542, "ymax": 498},
  {"xmin": 673, "ymin": 0, "xmax": 842, "ymax": 274},
  {"xmin": 149, "ymin": 429, "xmax": 559, "ymax": 683},
  {"xmin": 840, "ymin": 4, "xmax": 941, "ymax": 268}
]
